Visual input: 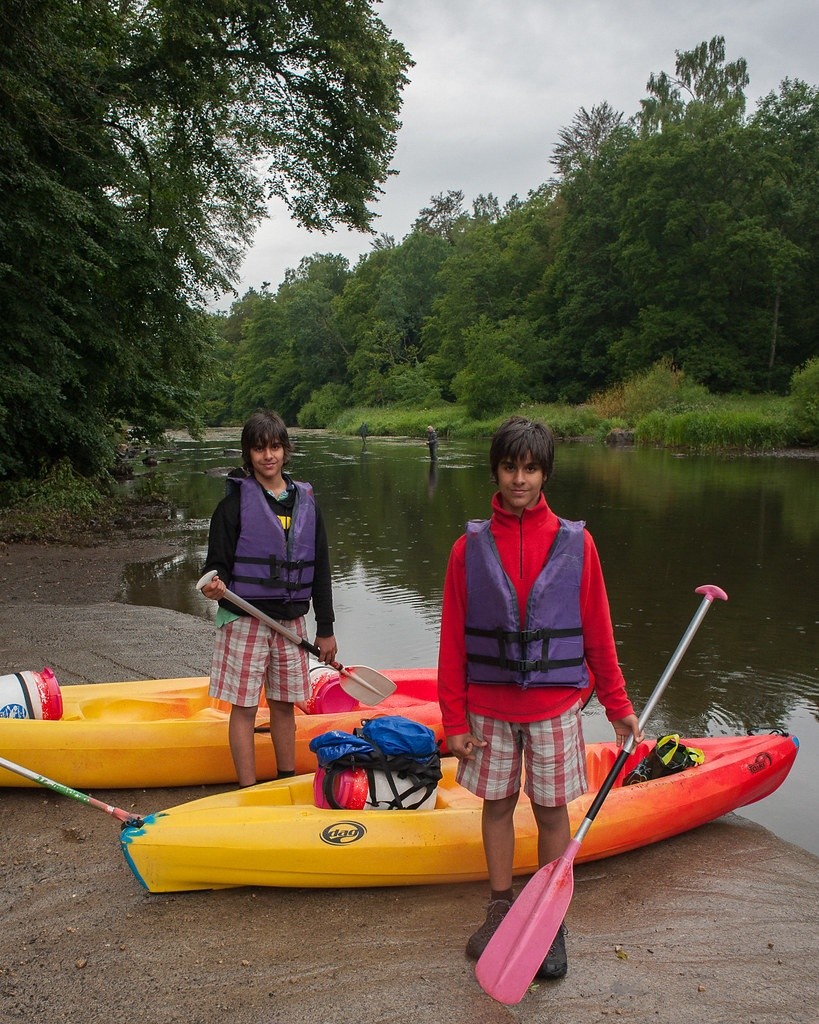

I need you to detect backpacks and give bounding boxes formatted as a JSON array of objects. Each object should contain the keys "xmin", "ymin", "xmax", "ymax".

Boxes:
[{"xmin": 308, "ymin": 715, "xmax": 442, "ymax": 809}]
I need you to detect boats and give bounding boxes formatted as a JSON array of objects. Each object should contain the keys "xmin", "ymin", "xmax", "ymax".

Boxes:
[
  {"xmin": 118, "ymin": 732, "xmax": 798, "ymax": 890},
  {"xmin": 0, "ymin": 666, "xmax": 454, "ymax": 789}
]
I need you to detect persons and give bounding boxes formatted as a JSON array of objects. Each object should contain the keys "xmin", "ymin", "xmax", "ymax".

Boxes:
[
  {"xmin": 426, "ymin": 426, "xmax": 437, "ymax": 461},
  {"xmin": 439, "ymin": 416, "xmax": 642, "ymax": 983},
  {"xmin": 360, "ymin": 422, "xmax": 368, "ymax": 445},
  {"xmin": 428, "ymin": 463, "xmax": 436, "ymax": 498},
  {"xmin": 196, "ymin": 412, "xmax": 338, "ymax": 790}
]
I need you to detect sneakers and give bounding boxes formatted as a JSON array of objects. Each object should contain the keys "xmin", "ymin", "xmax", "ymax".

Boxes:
[
  {"xmin": 465, "ymin": 887, "xmax": 515, "ymax": 958},
  {"xmin": 535, "ymin": 920, "xmax": 568, "ymax": 980}
]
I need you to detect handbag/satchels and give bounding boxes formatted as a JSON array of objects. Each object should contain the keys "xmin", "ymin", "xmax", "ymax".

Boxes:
[{"xmin": 621, "ymin": 733, "xmax": 704, "ymax": 787}]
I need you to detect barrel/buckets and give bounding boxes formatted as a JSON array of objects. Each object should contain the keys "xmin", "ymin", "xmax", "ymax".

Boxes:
[
  {"xmin": 312, "ymin": 763, "xmax": 437, "ymax": 809},
  {"xmin": 0, "ymin": 666, "xmax": 63, "ymax": 720},
  {"xmin": 294, "ymin": 658, "xmax": 355, "ymax": 715}
]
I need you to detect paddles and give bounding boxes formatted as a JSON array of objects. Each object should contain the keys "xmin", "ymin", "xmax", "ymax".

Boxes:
[
  {"xmin": 472, "ymin": 583, "xmax": 728, "ymax": 1008},
  {"xmin": 195, "ymin": 569, "xmax": 399, "ymax": 709}
]
